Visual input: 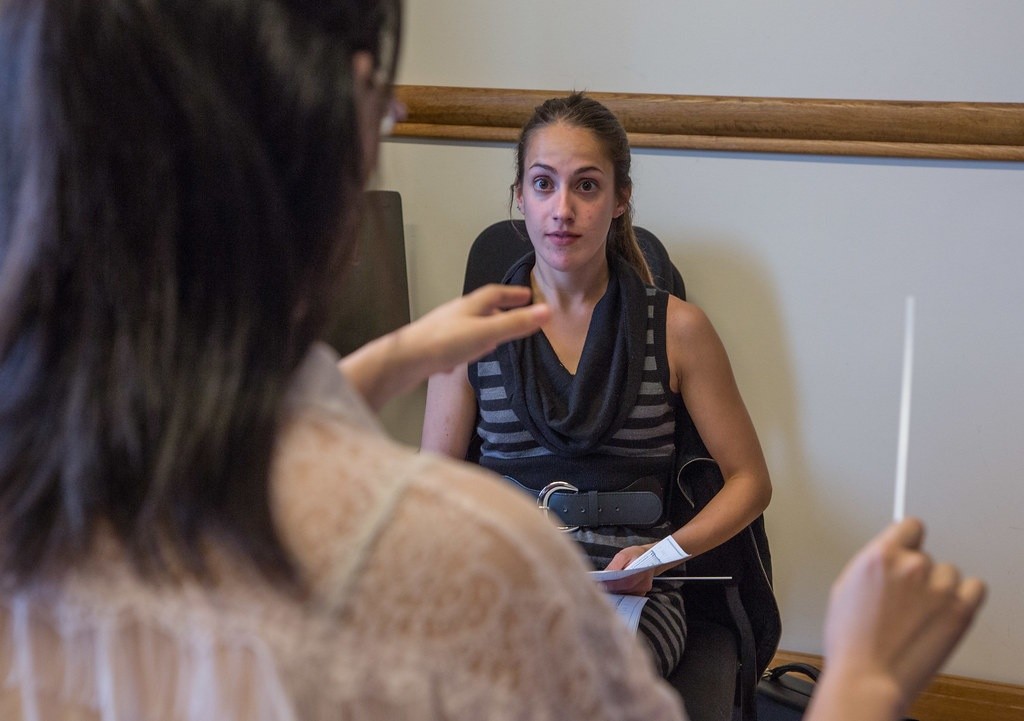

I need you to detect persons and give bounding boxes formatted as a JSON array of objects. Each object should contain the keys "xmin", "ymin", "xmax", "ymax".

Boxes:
[
  {"xmin": 0, "ymin": 0, "xmax": 988, "ymax": 720},
  {"xmin": 418, "ymin": 92, "xmax": 772, "ymax": 680}
]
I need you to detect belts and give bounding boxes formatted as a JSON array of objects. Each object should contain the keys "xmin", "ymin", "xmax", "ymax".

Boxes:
[{"xmin": 502, "ymin": 475, "xmax": 676, "ymax": 530}]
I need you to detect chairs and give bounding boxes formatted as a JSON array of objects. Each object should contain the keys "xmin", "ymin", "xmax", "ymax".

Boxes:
[{"xmin": 462, "ymin": 218, "xmax": 783, "ymax": 721}]
